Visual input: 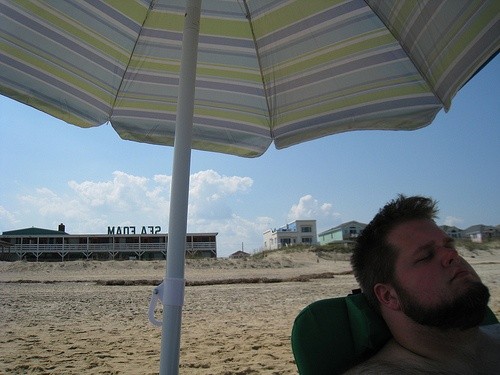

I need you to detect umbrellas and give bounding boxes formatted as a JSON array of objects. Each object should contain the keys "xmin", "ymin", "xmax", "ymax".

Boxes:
[{"xmin": 0, "ymin": 0, "xmax": 500, "ymax": 375}]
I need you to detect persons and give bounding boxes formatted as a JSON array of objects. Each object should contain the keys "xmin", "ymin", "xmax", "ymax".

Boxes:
[{"xmin": 344, "ymin": 194, "xmax": 500, "ymax": 375}]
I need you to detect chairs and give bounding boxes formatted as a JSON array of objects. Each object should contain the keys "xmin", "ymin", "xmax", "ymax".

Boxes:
[{"xmin": 292, "ymin": 288, "xmax": 499, "ymax": 375}]
